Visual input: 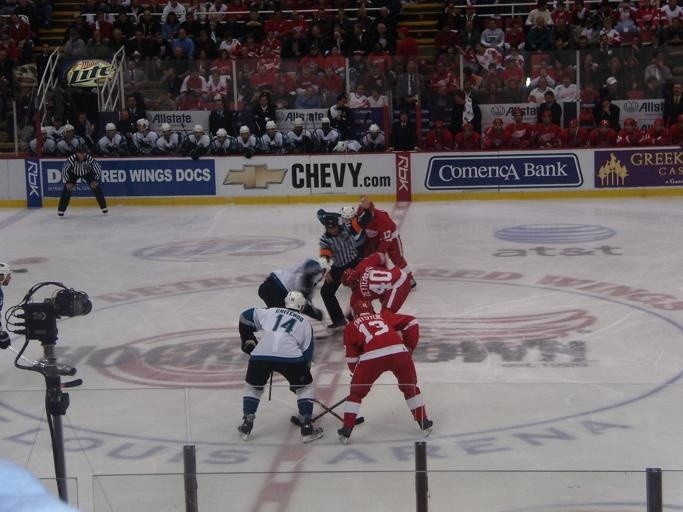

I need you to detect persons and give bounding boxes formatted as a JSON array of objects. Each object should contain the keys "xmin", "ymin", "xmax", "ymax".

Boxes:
[
  {"xmin": 0, "ymin": 259, "xmax": 13, "ymax": 350},
  {"xmin": 258, "ymin": 199, "xmax": 416, "ymax": 329},
  {"xmin": 392, "ymin": 27, "xmax": 424, "ymax": 152},
  {"xmin": 1, "ymin": 0, "xmax": 402, "ymax": 157},
  {"xmin": 236, "ymin": 292, "xmax": 324, "ymax": 439},
  {"xmin": 424, "ymin": 0, "xmax": 683, "ymax": 151},
  {"xmin": 54, "ymin": 143, "xmax": 109, "ymax": 217},
  {"xmin": 338, "ymin": 299, "xmax": 433, "ymax": 441}
]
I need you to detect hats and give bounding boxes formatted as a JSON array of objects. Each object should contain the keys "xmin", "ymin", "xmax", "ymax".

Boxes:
[{"xmin": 607, "ymin": 77, "xmax": 618, "ymax": 85}]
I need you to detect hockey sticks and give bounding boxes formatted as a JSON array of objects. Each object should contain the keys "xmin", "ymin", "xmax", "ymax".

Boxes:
[
  {"xmin": 313, "ymin": 396, "xmax": 365, "ymax": 425},
  {"xmin": 7, "ymin": 346, "xmax": 83, "ymax": 389},
  {"xmin": 291, "ymin": 397, "xmax": 348, "ymax": 426}
]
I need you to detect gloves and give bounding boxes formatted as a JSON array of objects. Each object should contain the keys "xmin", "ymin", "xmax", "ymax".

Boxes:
[
  {"xmin": 241, "ymin": 335, "xmax": 258, "ymax": 353},
  {"xmin": 0, "ymin": 331, "xmax": 10, "ymax": 349}
]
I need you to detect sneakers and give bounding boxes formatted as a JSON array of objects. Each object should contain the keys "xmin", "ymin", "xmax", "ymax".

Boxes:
[
  {"xmin": 58, "ymin": 211, "xmax": 64, "ymax": 216},
  {"xmin": 102, "ymin": 208, "xmax": 107, "ymax": 213},
  {"xmin": 327, "ymin": 320, "xmax": 347, "ymax": 328},
  {"xmin": 301, "ymin": 420, "xmax": 323, "ymax": 436},
  {"xmin": 337, "ymin": 427, "xmax": 353, "ymax": 437},
  {"xmin": 238, "ymin": 415, "xmax": 253, "ymax": 433},
  {"xmin": 417, "ymin": 417, "xmax": 433, "ymax": 430}
]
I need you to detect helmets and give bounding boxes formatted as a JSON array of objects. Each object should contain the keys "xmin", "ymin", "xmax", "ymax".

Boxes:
[
  {"xmin": 360, "ymin": 200, "xmax": 375, "ymax": 215},
  {"xmin": 316, "ymin": 256, "xmax": 333, "ymax": 275},
  {"xmin": 512, "ymin": 109, "xmax": 683, "ymax": 127},
  {"xmin": 341, "ymin": 268, "xmax": 360, "ymax": 286},
  {"xmin": 324, "ymin": 215, "xmax": 338, "ymax": 227},
  {"xmin": 137, "ymin": 119, "xmax": 149, "ymax": 131},
  {"xmin": 40, "ymin": 125, "xmax": 75, "ymax": 140},
  {"xmin": 161, "ymin": 117, "xmax": 330, "ymax": 137},
  {"xmin": 434, "ymin": 120, "xmax": 497, "ymax": 140},
  {"xmin": 340, "ymin": 206, "xmax": 355, "ymax": 219},
  {"xmin": 351, "ymin": 300, "xmax": 373, "ymax": 318},
  {"xmin": 76, "ymin": 144, "xmax": 88, "ymax": 158},
  {"xmin": 369, "ymin": 124, "xmax": 380, "ymax": 133},
  {"xmin": 106, "ymin": 123, "xmax": 116, "ymax": 130},
  {"xmin": 0, "ymin": 263, "xmax": 10, "ymax": 286},
  {"xmin": 284, "ymin": 291, "xmax": 306, "ymax": 312},
  {"xmin": 336, "ymin": 141, "xmax": 347, "ymax": 153}
]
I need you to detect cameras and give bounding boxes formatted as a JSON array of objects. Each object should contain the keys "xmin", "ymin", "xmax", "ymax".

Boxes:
[{"xmin": 44, "ymin": 290, "xmax": 92, "ymax": 318}]
